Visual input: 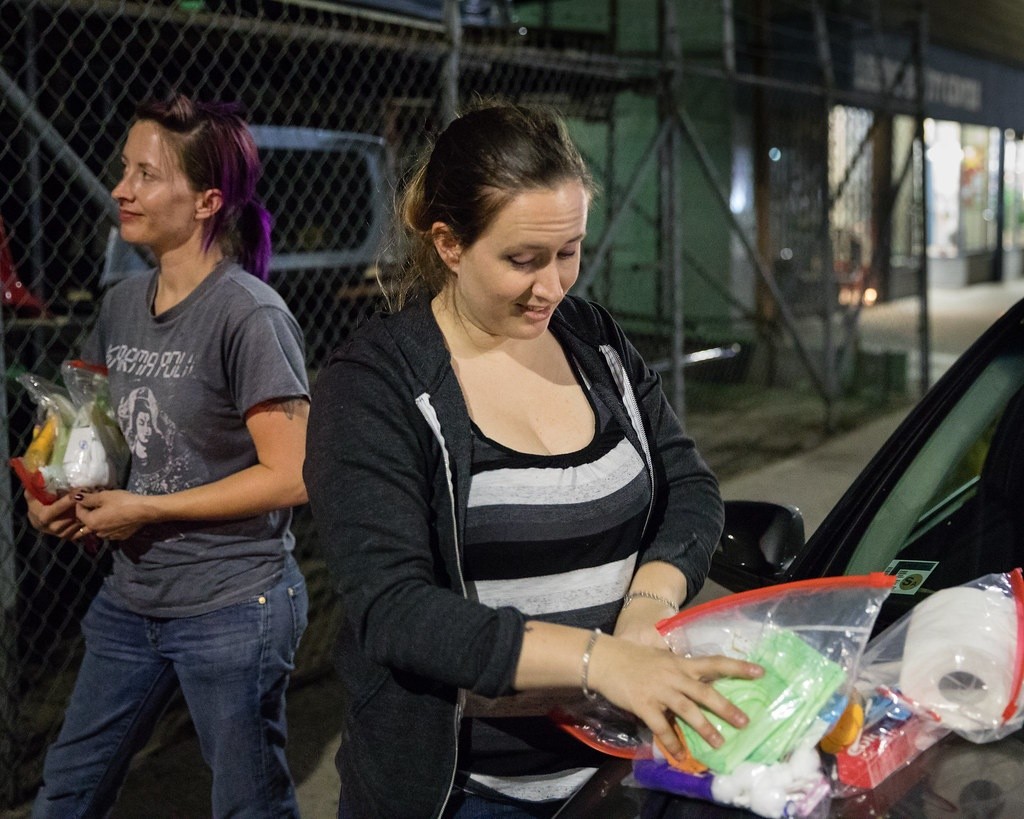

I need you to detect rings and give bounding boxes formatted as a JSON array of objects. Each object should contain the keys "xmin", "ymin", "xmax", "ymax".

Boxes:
[{"xmin": 80, "ymin": 527, "xmax": 86, "ymax": 536}]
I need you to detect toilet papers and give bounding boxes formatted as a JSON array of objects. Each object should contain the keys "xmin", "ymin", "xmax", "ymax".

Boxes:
[{"xmin": 900, "ymin": 585, "xmax": 1024, "ymax": 732}]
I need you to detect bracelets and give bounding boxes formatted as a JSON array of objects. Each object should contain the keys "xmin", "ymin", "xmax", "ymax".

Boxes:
[
  {"xmin": 623, "ymin": 591, "xmax": 681, "ymax": 613},
  {"xmin": 582, "ymin": 628, "xmax": 601, "ymax": 700}
]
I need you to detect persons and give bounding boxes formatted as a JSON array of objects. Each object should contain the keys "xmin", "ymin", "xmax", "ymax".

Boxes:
[
  {"xmin": 302, "ymin": 104, "xmax": 763, "ymax": 819},
  {"xmin": 25, "ymin": 93, "xmax": 312, "ymax": 819}
]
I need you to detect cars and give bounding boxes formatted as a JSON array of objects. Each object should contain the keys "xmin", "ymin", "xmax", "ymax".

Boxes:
[{"xmin": 550, "ymin": 297, "xmax": 1024, "ymax": 819}]
